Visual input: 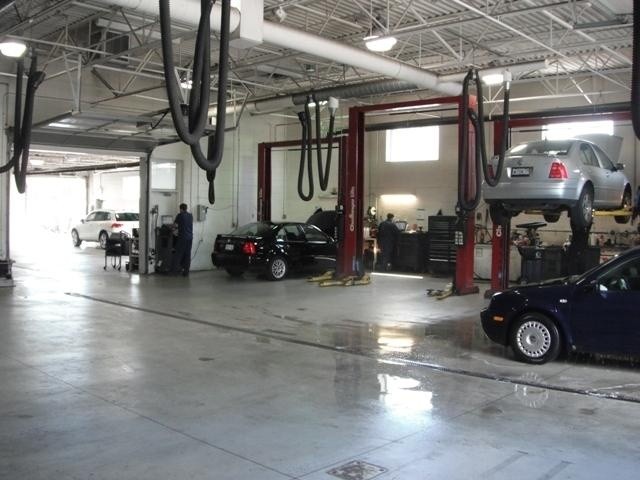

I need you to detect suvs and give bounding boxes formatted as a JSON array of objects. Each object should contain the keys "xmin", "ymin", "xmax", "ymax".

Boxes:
[{"xmin": 70, "ymin": 208, "xmax": 139, "ymax": 250}]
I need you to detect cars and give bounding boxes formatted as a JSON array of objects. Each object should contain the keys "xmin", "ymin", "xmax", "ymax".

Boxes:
[
  {"xmin": 475, "ymin": 244, "xmax": 640, "ymax": 370},
  {"xmin": 481, "ymin": 134, "xmax": 635, "ymax": 229},
  {"xmin": 210, "ymin": 207, "xmax": 341, "ymax": 281}
]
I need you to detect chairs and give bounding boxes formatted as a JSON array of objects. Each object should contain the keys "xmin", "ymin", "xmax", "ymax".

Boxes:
[{"xmin": 286, "ymin": 232, "xmax": 297, "ymax": 241}]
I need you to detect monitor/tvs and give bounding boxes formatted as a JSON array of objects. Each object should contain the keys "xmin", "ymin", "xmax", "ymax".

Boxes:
[{"xmin": 396, "ymin": 221, "xmax": 406, "ymax": 232}]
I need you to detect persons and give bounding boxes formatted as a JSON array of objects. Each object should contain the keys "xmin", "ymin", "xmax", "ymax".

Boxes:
[
  {"xmin": 379, "ymin": 214, "xmax": 399, "ymax": 271},
  {"xmin": 174, "ymin": 204, "xmax": 193, "ymax": 275}
]
